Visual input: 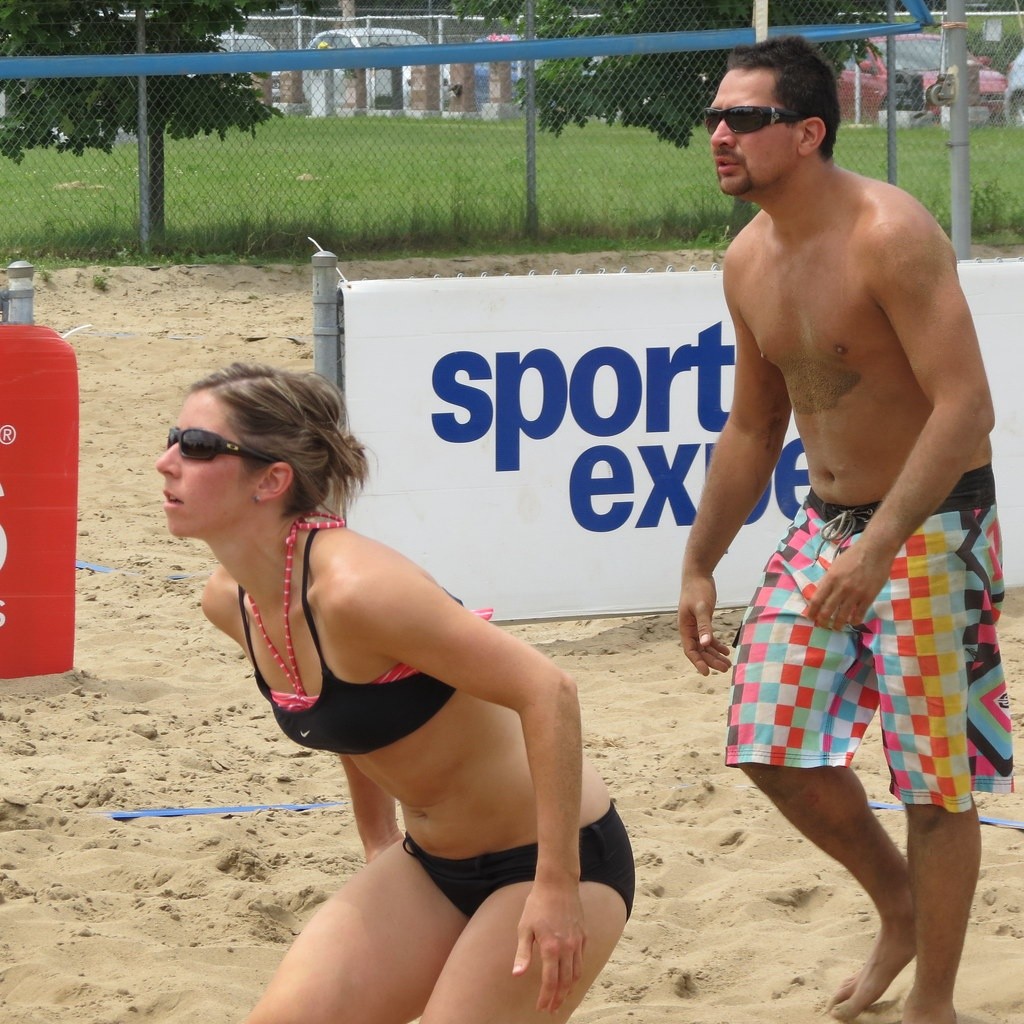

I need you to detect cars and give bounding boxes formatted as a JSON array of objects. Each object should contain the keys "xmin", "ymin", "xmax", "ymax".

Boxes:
[
  {"xmin": 210, "ymin": 30, "xmax": 282, "ymax": 102},
  {"xmin": 454, "ymin": 33, "xmax": 594, "ymax": 102},
  {"xmin": 840, "ymin": 32, "xmax": 1007, "ymax": 127},
  {"xmin": 300, "ymin": 28, "xmax": 453, "ymax": 110},
  {"xmin": 1002, "ymin": 46, "xmax": 1024, "ymax": 126}
]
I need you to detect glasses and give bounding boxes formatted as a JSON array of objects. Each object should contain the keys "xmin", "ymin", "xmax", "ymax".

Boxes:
[
  {"xmin": 703, "ymin": 105, "xmax": 802, "ymax": 135},
  {"xmin": 168, "ymin": 426, "xmax": 287, "ymax": 463}
]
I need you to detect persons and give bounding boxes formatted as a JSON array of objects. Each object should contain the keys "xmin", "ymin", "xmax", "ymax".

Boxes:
[
  {"xmin": 156, "ymin": 364, "xmax": 634, "ymax": 1024},
  {"xmin": 678, "ymin": 34, "xmax": 1021, "ymax": 1024}
]
